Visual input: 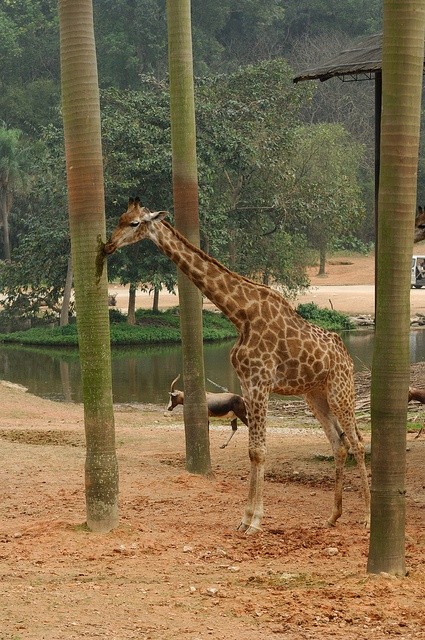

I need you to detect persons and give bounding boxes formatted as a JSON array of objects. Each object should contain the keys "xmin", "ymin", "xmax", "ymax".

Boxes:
[{"xmin": 422, "ymin": 262, "xmax": 425, "ymax": 276}]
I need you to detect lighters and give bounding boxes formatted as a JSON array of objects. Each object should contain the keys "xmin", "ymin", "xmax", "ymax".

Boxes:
[{"xmin": 103, "ymin": 196, "xmax": 371, "ymax": 536}]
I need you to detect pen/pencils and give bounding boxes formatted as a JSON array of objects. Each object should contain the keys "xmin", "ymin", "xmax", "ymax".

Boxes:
[{"xmin": 411, "ymin": 255, "xmax": 425, "ymax": 289}]
